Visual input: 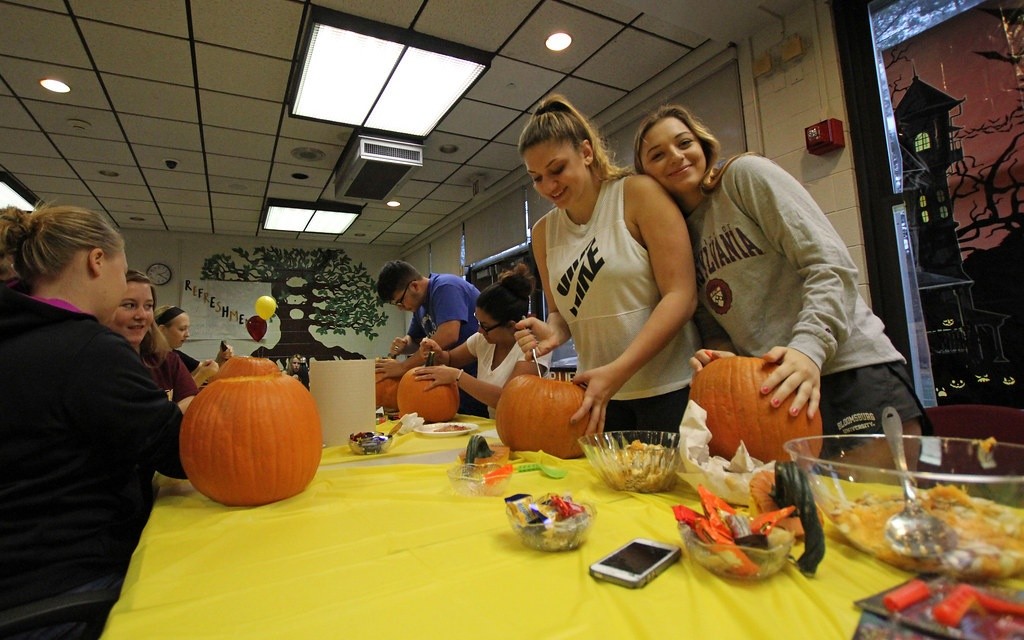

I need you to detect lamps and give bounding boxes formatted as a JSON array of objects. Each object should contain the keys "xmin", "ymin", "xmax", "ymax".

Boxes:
[
  {"xmin": 0, "ymin": 171, "xmax": 41, "ymax": 213},
  {"xmin": 262, "ymin": 198, "xmax": 362, "ymax": 235},
  {"xmin": 288, "ymin": 5, "xmax": 491, "ymax": 141}
]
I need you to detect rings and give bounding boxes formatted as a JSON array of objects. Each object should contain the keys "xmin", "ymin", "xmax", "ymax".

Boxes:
[{"xmin": 393, "ymin": 346, "xmax": 398, "ymax": 351}]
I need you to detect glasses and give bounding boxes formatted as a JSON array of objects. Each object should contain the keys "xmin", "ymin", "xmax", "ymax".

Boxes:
[
  {"xmin": 473, "ymin": 311, "xmax": 507, "ymax": 335},
  {"xmin": 392, "ymin": 279, "xmax": 417, "ymax": 307}
]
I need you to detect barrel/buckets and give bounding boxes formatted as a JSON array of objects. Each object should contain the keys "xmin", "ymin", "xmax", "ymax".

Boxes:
[{"xmin": 307, "ymin": 357, "xmax": 376, "ymax": 448}]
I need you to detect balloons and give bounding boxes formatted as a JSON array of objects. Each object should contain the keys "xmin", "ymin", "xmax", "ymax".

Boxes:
[
  {"xmin": 247, "ymin": 315, "xmax": 267, "ymax": 343},
  {"xmin": 255, "ymin": 295, "xmax": 277, "ymax": 322}
]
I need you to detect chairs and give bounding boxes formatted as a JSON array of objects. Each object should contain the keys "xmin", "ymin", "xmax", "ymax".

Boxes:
[{"xmin": 920, "ymin": 406, "xmax": 1023, "ymax": 475}]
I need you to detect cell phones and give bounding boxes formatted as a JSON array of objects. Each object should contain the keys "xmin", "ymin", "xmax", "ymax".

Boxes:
[{"xmin": 589, "ymin": 538, "xmax": 681, "ymax": 589}]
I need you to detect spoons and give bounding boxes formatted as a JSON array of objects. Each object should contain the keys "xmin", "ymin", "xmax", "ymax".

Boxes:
[{"xmin": 881, "ymin": 407, "xmax": 955, "ymax": 558}]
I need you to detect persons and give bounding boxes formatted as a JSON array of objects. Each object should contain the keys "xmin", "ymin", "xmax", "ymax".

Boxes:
[
  {"xmin": 374, "ymin": 259, "xmax": 490, "ymax": 420},
  {"xmin": 511, "ymin": 96, "xmax": 704, "ymax": 444},
  {"xmin": 0, "ymin": 197, "xmax": 190, "ymax": 640},
  {"xmin": 282, "ymin": 353, "xmax": 310, "ymax": 392},
  {"xmin": 103, "ymin": 265, "xmax": 234, "ymax": 414},
  {"xmin": 633, "ymin": 108, "xmax": 928, "ymax": 487},
  {"xmin": 413, "ymin": 262, "xmax": 553, "ymax": 419}
]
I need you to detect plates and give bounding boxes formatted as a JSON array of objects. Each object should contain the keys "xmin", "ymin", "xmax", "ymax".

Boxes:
[{"xmin": 413, "ymin": 422, "xmax": 479, "ymax": 437}]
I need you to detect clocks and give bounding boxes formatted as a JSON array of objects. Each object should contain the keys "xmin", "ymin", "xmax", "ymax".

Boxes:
[{"xmin": 146, "ymin": 263, "xmax": 171, "ymax": 285}]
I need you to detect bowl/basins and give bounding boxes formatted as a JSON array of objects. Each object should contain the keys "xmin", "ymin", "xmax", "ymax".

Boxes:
[
  {"xmin": 350, "ymin": 436, "xmax": 393, "ymax": 455},
  {"xmin": 782, "ymin": 434, "xmax": 1024, "ymax": 584},
  {"xmin": 577, "ymin": 431, "xmax": 683, "ymax": 493},
  {"xmin": 676, "ymin": 520, "xmax": 793, "ymax": 581},
  {"xmin": 507, "ymin": 501, "xmax": 596, "ymax": 551},
  {"xmin": 446, "ymin": 464, "xmax": 512, "ymax": 497}
]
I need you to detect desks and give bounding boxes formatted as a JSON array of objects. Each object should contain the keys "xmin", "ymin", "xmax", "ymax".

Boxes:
[{"xmin": 99, "ymin": 415, "xmax": 1024, "ymax": 640}]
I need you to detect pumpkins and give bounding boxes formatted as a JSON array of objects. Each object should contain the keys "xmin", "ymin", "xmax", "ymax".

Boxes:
[
  {"xmin": 207, "ymin": 356, "xmax": 281, "ymax": 382},
  {"xmin": 375, "ymin": 372, "xmax": 401, "ymax": 411},
  {"xmin": 397, "ymin": 351, "xmax": 460, "ymax": 423},
  {"xmin": 179, "ymin": 373, "xmax": 322, "ymax": 506},
  {"xmin": 688, "ymin": 356, "xmax": 823, "ymax": 472},
  {"xmin": 495, "ymin": 374, "xmax": 597, "ymax": 459}
]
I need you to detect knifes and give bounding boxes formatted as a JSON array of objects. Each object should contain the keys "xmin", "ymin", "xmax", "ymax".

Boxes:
[{"xmin": 521, "ymin": 314, "xmax": 543, "ymax": 378}]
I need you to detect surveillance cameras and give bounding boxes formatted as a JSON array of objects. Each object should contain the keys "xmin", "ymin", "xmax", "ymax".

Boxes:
[{"xmin": 165, "ymin": 160, "xmax": 177, "ymax": 169}]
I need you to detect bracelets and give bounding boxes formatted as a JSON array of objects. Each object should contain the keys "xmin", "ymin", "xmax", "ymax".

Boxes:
[{"xmin": 453, "ymin": 369, "xmax": 464, "ymax": 386}]
